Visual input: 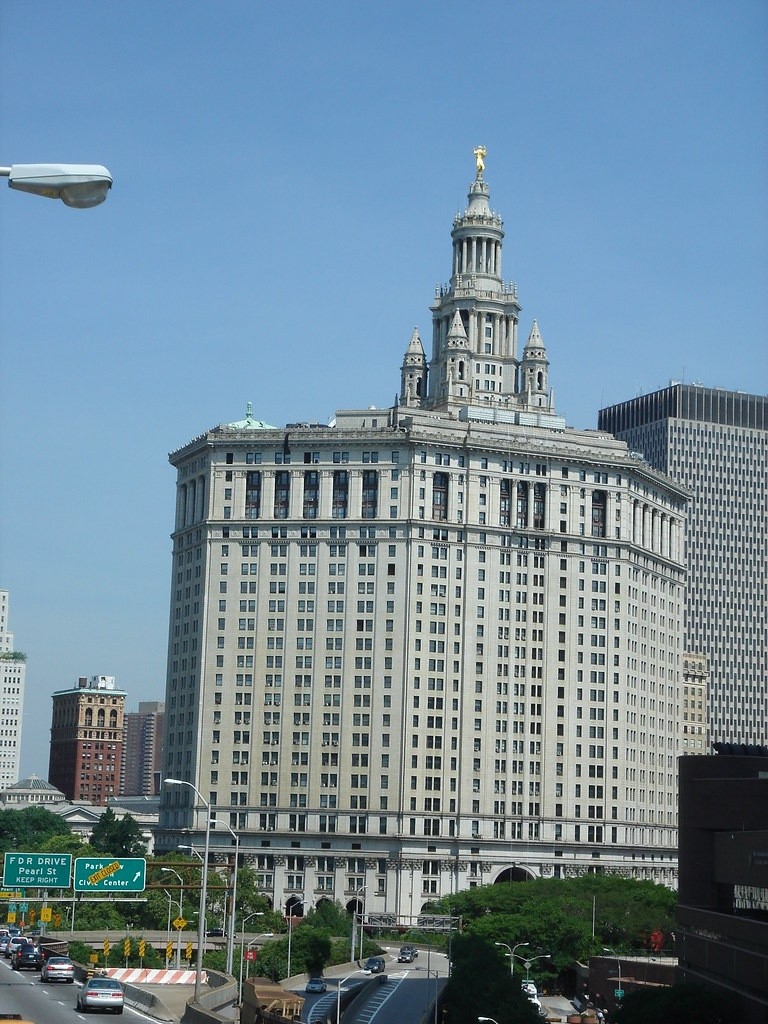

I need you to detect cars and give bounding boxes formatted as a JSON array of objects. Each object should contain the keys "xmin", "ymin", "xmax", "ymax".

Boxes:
[
  {"xmin": 76, "ymin": 977, "xmax": 125, "ymax": 1015},
  {"xmin": 41, "ymin": 956, "xmax": 75, "ymax": 984},
  {"xmin": 202, "ymin": 927, "xmax": 236, "ymax": 939},
  {"xmin": 0, "ymin": 928, "xmax": 22, "ymax": 954},
  {"xmin": 398, "ymin": 945, "xmax": 419, "ymax": 964},
  {"xmin": 6, "ymin": 936, "xmax": 29, "ymax": 958},
  {"xmin": 305, "ymin": 978, "xmax": 328, "ymax": 993}
]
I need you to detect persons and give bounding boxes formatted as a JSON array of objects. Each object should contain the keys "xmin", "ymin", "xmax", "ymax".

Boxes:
[{"xmin": 596, "ymin": 1011, "xmax": 603, "ymax": 1024}]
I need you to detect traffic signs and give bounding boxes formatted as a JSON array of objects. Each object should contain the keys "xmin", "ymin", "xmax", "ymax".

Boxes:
[{"xmin": 73, "ymin": 857, "xmax": 146, "ymax": 892}]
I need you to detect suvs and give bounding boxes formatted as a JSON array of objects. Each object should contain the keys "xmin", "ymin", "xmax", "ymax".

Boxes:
[
  {"xmin": 364, "ymin": 957, "xmax": 386, "ymax": 973},
  {"xmin": 11, "ymin": 944, "xmax": 45, "ymax": 970}
]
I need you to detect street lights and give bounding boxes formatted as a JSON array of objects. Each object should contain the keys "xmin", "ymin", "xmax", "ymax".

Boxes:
[
  {"xmin": 495, "ymin": 941, "xmax": 529, "ymax": 978},
  {"xmin": 205, "ymin": 819, "xmax": 239, "ymax": 976},
  {"xmin": 193, "ymin": 912, "xmax": 207, "ymax": 956},
  {"xmin": 603, "ymin": 948, "xmax": 624, "ymax": 1006},
  {"xmin": 238, "ymin": 912, "xmax": 264, "ymax": 1002},
  {"xmin": 287, "ymin": 900, "xmax": 308, "ymax": 979},
  {"xmin": 504, "ymin": 954, "xmax": 550, "ymax": 984},
  {"xmin": 356, "ymin": 886, "xmax": 369, "ymax": 914},
  {"xmin": 163, "ymin": 778, "xmax": 212, "ymax": 1001},
  {"xmin": 415, "ymin": 966, "xmax": 439, "ymax": 1024},
  {"xmin": 246, "ymin": 932, "xmax": 274, "ymax": 981},
  {"xmin": 178, "ymin": 845, "xmax": 203, "ymax": 919},
  {"xmin": 336, "ymin": 970, "xmax": 372, "ymax": 1023},
  {"xmin": 161, "ymin": 868, "xmax": 183, "ymax": 969}
]
